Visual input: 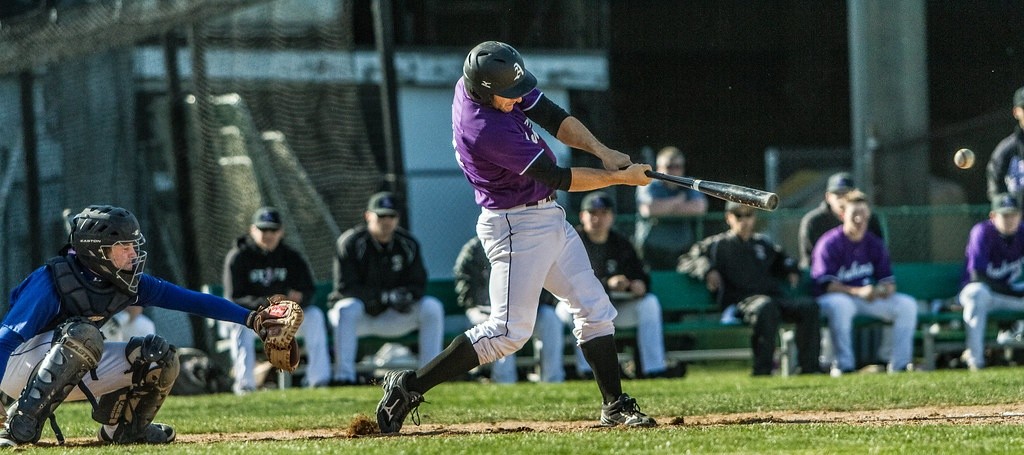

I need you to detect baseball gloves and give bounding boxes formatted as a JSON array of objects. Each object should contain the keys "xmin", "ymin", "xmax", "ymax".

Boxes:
[{"xmin": 253, "ymin": 300, "xmax": 305, "ymax": 372}]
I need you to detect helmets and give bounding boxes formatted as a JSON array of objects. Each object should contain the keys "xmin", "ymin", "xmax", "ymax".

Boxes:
[
  {"xmin": 463, "ymin": 41, "xmax": 537, "ymax": 109},
  {"xmin": 69, "ymin": 204, "xmax": 147, "ymax": 299}
]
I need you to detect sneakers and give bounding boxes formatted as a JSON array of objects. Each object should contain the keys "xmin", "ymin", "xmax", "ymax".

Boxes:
[
  {"xmin": 601, "ymin": 393, "xmax": 657, "ymax": 429},
  {"xmin": 97, "ymin": 422, "xmax": 176, "ymax": 444},
  {"xmin": 376, "ymin": 369, "xmax": 431, "ymax": 436},
  {"xmin": 0, "ymin": 423, "xmax": 17, "ymax": 447}
]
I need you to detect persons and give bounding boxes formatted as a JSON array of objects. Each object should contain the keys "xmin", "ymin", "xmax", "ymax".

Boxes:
[
  {"xmin": 327, "ymin": 192, "xmax": 445, "ymax": 387},
  {"xmin": 678, "ymin": 201, "xmax": 825, "ymax": 377},
  {"xmin": 555, "ymin": 192, "xmax": 685, "ymax": 380},
  {"xmin": 798, "ymin": 173, "xmax": 885, "ymax": 298},
  {"xmin": 0, "ymin": 205, "xmax": 304, "ymax": 449},
  {"xmin": 219, "ymin": 206, "xmax": 331, "ymax": 396},
  {"xmin": 811, "ymin": 189, "xmax": 917, "ymax": 374},
  {"xmin": 453, "ymin": 235, "xmax": 564, "ymax": 383},
  {"xmin": 959, "ymin": 193, "xmax": 1024, "ymax": 370},
  {"xmin": 634, "ymin": 146, "xmax": 708, "ymax": 365},
  {"xmin": 987, "ymin": 87, "xmax": 1024, "ymax": 219},
  {"xmin": 376, "ymin": 41, "xmax": 656, "ymax": 436}
]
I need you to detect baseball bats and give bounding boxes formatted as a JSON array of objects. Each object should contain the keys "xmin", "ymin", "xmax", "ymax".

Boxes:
[{"xmin": 619, "ymin": 164, "xmax": 780, "ymax": 213}]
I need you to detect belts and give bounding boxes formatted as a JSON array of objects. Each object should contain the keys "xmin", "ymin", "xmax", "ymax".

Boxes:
[{"xmin": 525, "ymin": 194, "xmax": 556, "ymax": 207}]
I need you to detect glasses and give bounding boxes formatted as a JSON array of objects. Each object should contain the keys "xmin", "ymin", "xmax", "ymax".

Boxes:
[
  {"xmin": 377, "ymin": 213, "xmax": 398, "ymax": 220},
  {"xmin": 257, "ymin": 228, "xmax": 280, "ymax": 232},
  {"xmin": 732, "ymin": 210, "xmax": 754, "ymax": 217}
]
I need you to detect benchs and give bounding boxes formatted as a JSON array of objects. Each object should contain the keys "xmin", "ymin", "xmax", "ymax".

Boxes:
[{"xmin": 200, "ymin": 260, "xmax": 1024, "ymax": 389}]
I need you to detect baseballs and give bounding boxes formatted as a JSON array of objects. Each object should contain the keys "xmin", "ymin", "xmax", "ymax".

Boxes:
[{"xmin": 953, "ymin": 148, "xmax": 976, "ymax": 169}]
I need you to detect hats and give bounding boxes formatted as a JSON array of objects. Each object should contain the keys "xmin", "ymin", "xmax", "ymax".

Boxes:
[
  {"xmin": 1013, "ymin": 87, "xmax": 1024, "ymax": 107},
  {"xmin": 367, "ymin": 191, "xmax": 402, "ymax": 216},
  {"xmin": 825, "ymin": 173, "xmax": 856, "ymax": 194},
  {"xmin": 991, "ymin": 192, "xmax": 1024, "ymax": 210},
  {"xmin": 253, "ymin": 206, "xmax": 285, "ymax": 228},
  {"xmin": 724, "ymin": 198, "xmax": 752, "ymax": 208},
  {"xmin": 580, "ymin": 191, "xmax": 616, "ymax": 212}
]
